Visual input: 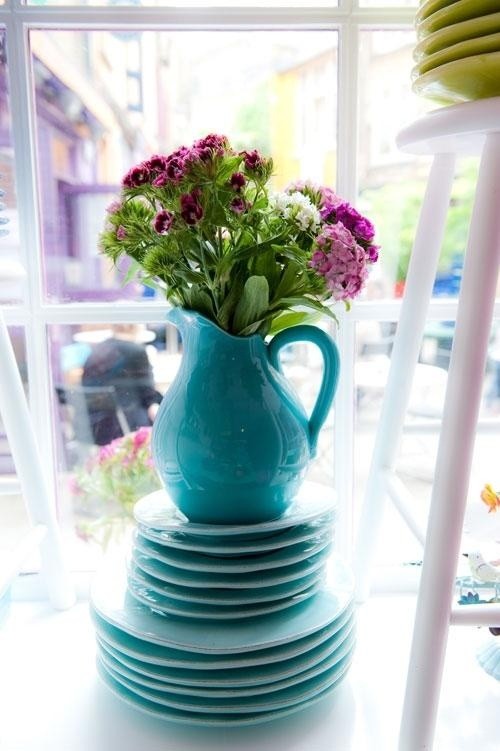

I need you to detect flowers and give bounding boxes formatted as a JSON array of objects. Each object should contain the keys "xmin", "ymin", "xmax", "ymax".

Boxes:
[
  {"xmin": 66, "ymin": 426, "xmax": 152, "ymax": 547},
  {"xmin": 96, "ymin": 132, "xmax": 381, "ymax": 335}
]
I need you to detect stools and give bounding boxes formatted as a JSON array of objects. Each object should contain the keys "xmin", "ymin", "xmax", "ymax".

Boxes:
[{"xmin": 355, "ymin": 97, "xmax": 499, "ymax": 747}]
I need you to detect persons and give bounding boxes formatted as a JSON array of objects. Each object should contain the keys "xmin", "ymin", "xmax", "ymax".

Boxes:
[{"xmin": 82, "ymin": 324, "xmax": 167, "ymax": 445}]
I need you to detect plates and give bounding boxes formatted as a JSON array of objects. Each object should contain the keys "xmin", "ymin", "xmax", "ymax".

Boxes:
[
  {"xmin": 410, "ymin": 0, "xmax": 500, "ymax": 105},
  {"xmin": 84, "ymin": 558, "xmax": 357, "ymax": 729},
  {"xmin": 126, "ymin": 491, "xmax": 337, "ymax": 623}
]
